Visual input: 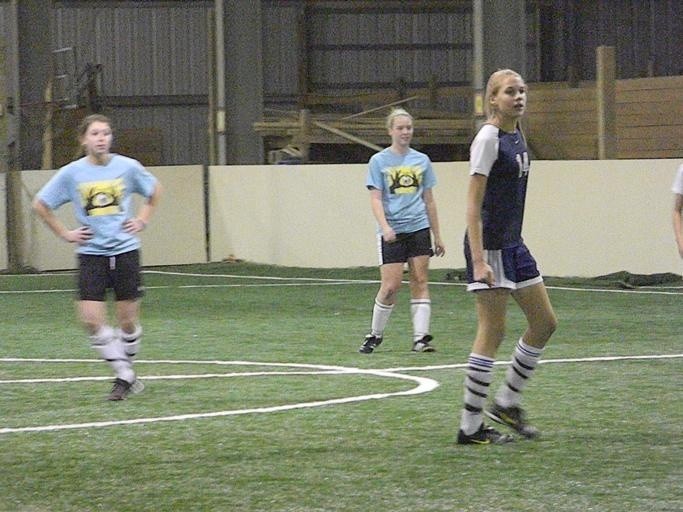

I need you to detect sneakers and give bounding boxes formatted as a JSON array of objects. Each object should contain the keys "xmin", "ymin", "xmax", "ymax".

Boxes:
[
  {"xmin": 458, "ymin": 423, "xmax": 511, "ymax": 444},
  {"xmin": 108, "ymin": 376, "xmax": 145, "ymax": 400},
  {"xmin": 412, "ymin": 335, "xmax": 435, "ymax": 352},
  {"xmin": 485, "ymin": 400, "xmax": 542, "ymax": 440},
  {"xmin": 359, "ymin": 334, "xmax": 383, "ymax": 355}
]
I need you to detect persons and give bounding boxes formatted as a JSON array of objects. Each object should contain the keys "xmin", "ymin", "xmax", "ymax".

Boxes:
[
  {"xmin": 671, "ymin": 162, "xmax": 682, "ymax": 261},
  {"xmin": 456, "ymin": 68, "xmax": 557, "ymax": 447},
  {"xmin": 27, "ymin": 112, "xmax": 161, "ymax": 403},
  {"xmin": 356, "ymin": 106, "xmax": 445, "ymax": 354}
]
